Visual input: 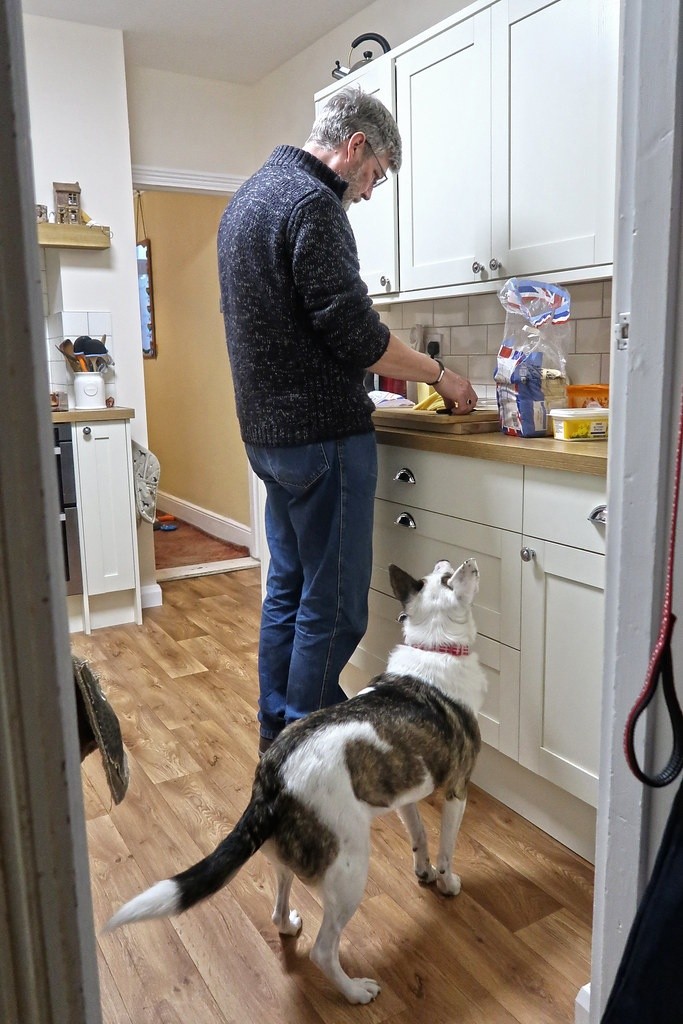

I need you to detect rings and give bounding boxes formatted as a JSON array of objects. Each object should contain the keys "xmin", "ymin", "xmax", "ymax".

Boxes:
[{"xmin": 465, "ymin": 399, "xmax": 472, "ymax": 405}]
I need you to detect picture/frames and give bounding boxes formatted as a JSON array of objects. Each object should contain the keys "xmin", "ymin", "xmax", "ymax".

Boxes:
[{"xmin": 135, "ymin": 240, "xmax": 158, "ymax": 360}]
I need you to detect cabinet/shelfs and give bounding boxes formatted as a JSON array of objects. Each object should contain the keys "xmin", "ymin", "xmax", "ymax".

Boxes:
[
  {"xmin": 311, "ymin": 0, "xmax": 616, "ymax": 295},
  {"xmin": 48, "ymin": 402, "xmax": 143, "ymax": 636},
  {"xmin": 348, "ymin": 401, "xmax": 608, "ymax": 874}
]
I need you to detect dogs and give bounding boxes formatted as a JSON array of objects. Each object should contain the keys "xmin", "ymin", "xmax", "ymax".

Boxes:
[{"xmin": 104, "ymin": 558, "xmax": 483, "ymax": 1004}]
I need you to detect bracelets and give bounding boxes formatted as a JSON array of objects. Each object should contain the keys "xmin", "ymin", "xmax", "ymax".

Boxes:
[{"xmin": 426, "ymin": 360, "xmax": 445, "ymax": 385}]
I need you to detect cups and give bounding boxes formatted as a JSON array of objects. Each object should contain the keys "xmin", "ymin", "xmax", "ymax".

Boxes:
[
  {"xmin": 378, "ymin": 373, "xmax": 408, "ymax": 401},
  {"xmin": 72, "ymin": 372, "xmax": 107, "ymax": 410}
]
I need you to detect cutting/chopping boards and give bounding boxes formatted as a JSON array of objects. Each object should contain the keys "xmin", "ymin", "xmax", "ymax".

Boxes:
[{"xmin": 370, "ymin": 407, "xmax": 501, "ymax": 424}]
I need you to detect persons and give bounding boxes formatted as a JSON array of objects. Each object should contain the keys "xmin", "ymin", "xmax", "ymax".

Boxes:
[{"xmin": 217, "ymin": 87, "xmax": 479, "ymax": 760}]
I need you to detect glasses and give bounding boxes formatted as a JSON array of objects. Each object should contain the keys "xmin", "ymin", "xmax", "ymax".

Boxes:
[{"xmin": 348, "ymin": 133, "xmax": 388, "ymax": 188}]
